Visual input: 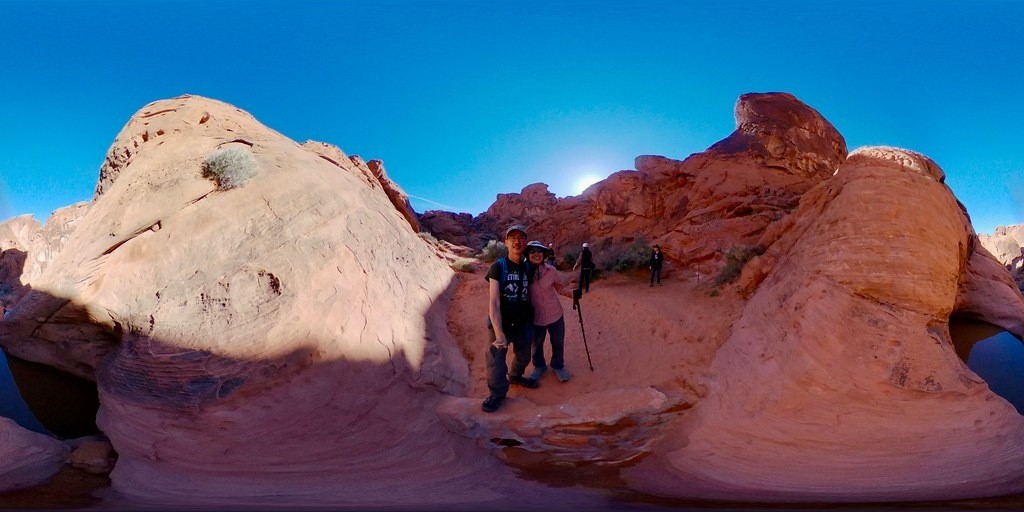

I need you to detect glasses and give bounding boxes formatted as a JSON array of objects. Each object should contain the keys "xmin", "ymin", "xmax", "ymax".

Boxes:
[{"xmin": 654, "ymin": 246, "xmax": 658, "ymax": 248}]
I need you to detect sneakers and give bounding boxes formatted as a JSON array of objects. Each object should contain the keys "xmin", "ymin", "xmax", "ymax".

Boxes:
[
  {"xmin": 482, "ymin": 396, "xmax": 503, "ymax": 411},
  {"xmin": 529, "ymin": 366, "xmax": 569, "ymax": 382},
  {"xmin": 507, "ymin": 372, "xmax": 538, "ymax": 388}
]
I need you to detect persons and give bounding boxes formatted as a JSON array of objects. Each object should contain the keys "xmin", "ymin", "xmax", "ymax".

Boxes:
[
  {"xmin": 481, "ymin": 225, "xmax": 539, "ymax": 412},
  {"xmin": 573, "ymin": 243, "xmax": 592, "ymax": 294},
  {"xmin": 547, "ymin": 243, "xmax": 557, "ymax": 267},
  {"xmin": 649, "ymin": 244, "xmax": 664, "ymax": 287},
  {"xmin": 524, "ymin": 240, "xmax": 583, "ymax": 382}
]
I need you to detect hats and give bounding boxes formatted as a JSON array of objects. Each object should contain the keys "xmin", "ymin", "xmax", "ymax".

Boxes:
[
  {"xmin": 583, "ymin": 243, "xmax": 589, "ymax": 247},
  {"xmin": 523, "ymin": 241, "xmax": 550, "ymax": 258},
  {"xmin": 506, "ymin": 226, "xmax": 527, "ymax": 237}
]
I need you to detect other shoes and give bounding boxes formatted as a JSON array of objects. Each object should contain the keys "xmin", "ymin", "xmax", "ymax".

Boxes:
[{"xmin": 650, "ymin": 284, "xmax": 663, "ymax": 288}]
